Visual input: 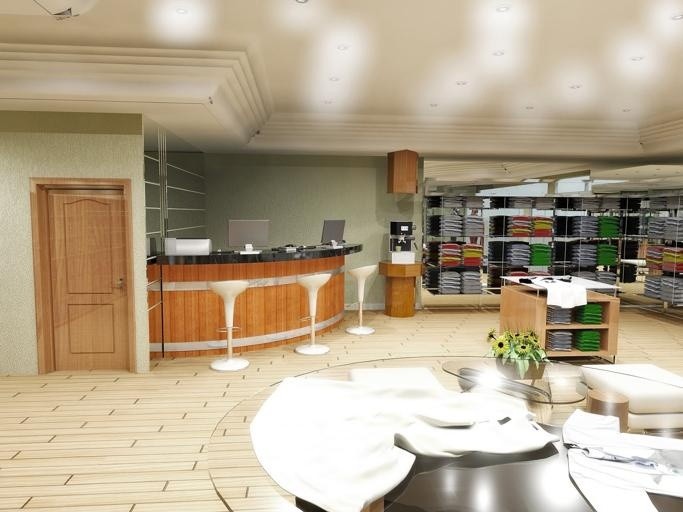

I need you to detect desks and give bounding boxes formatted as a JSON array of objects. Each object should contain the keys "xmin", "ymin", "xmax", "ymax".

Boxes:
[
  {"xmin": 161, "ymin": 241, "xmax": 363, "ymax": 357},
  {"xmin": 379, "ymin": 260, "xmax": 424, "ymax": 318},
  {"xmin": 206, "ymin": 356, "xmax": 683, "ymax": 512}
]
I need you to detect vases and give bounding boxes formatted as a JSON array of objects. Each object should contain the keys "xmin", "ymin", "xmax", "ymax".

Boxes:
[{"xmin": 495, "ymin": 360, "xmax": 546, "ymax": 380}]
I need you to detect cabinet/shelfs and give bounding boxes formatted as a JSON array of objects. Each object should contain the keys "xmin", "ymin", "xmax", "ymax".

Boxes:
[
  {"xmin": 497, "ymin": 272, "xmax": 621, "ymax": 363},
  {"xmin": 622, "ymin": 197, "xmax": 683, "ymax": 325},
  {"xmin": 420, "ymin": 179, "xmax": 630, "ymax": 311}
]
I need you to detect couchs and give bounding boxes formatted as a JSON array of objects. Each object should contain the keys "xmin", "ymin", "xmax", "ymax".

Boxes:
[{"xmin": 579, "ymin": 363, "xmax": 683, "ymax": 429}]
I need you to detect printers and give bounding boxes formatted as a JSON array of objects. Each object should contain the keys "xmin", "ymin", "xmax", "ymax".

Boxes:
[{"xmin": 163, "ymin": 236, "xmax": 213, "ymax": 255}]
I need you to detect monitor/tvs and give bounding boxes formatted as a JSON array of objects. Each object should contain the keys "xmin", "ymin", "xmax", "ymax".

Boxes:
[
  {"xmin": 227, "ymin": 219, "xmax": 270, "ymax": 255},
  {"xmin": 320, "ymin": 219, "xmax": 346, "ymax": 250}
]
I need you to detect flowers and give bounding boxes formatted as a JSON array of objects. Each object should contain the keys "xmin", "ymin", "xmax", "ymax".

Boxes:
[{"xmin": 484, "ymin": 329, "xmax": 551, "ymax": 381}]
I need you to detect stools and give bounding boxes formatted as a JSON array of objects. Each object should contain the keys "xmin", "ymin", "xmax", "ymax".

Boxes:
[
  {"xmin": 294, "ymin": 274, "xmax": 333, "ymax": 355},
  {"xmin": 346, "ymin": 263, "xmax": 375, "ymax": 335},
  {"xmin": 586, "ymin": 390, "xmax": 629, "ymax": 434},
  {"xmin": 207, "ymin": 281, "xmax": 252, "ymax": 372}
]
